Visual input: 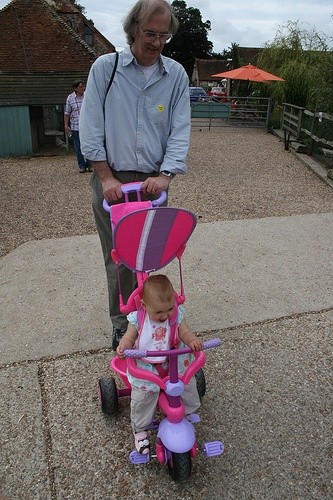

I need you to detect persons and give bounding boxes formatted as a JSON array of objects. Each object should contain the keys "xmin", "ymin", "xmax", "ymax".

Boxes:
[
  {"xmin": 65, "ymin": 79, "xmax": 93, "ymax": 173},
  {"xmin": 206, "ymin": 87, "xmax": 225, "ymax": 95},
  {"xmin": 232, "ymin": 98, "xmax": 241, "ymax": 108},
  {"xmin": 79, "ymin": 0, "xmax": 191, "ymax": 351},
  {"xmin": 117, "ymin": 274, "xmax": 204, "ymax": 455}
]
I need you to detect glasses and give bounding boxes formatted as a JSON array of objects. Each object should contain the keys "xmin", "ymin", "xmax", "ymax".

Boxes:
[{"xmin": 140, "ymin": 28, "xmax": 172, "ymax": 44}]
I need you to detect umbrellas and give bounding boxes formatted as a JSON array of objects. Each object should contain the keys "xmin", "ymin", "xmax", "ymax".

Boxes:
[{"xmin": 210, "ymin": 64, "xmax": 286, "ymax": 101}]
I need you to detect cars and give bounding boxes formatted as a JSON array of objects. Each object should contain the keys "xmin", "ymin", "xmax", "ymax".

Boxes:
[
  {"xmin": 209, "ymin": 87, "xmax": 227, "ymax": 102},
  {"xmin": 189, "ymin": 87, "xmax": 210, "ymax": 102},
  {"xmin": 201, "ymin": 86, "xmax": 212, "ymax": 94}
]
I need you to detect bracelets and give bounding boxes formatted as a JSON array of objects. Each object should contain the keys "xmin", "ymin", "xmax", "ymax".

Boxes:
[{"xmin": 160, "ymin": 170, "xmax": 174, "ymax": 179}]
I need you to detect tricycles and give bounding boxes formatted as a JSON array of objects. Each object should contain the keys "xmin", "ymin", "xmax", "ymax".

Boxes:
[{"xmin": 96, "ymin": 181, "xmax": 224, "ymax": 484}]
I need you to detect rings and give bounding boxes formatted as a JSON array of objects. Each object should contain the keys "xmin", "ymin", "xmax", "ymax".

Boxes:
[{"xmin": 152, "ymin": 188, "xmax": 156, "ymax": 191}]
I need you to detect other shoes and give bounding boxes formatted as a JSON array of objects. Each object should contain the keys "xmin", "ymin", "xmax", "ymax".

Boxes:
[
  {"xmin": 111, "ymin": 324, "xmax": 128, "ymax": 351},
  {"xmin": 79, "ymin": 168, "xmax": 86, "ymax": 173},
  {"xmin": 88, "ymin": 165, "xmax": 93, "ymax": 172},
  {"xmin": 133, "ymin": 429, "xmax": 152, "ymax": 456}
]
